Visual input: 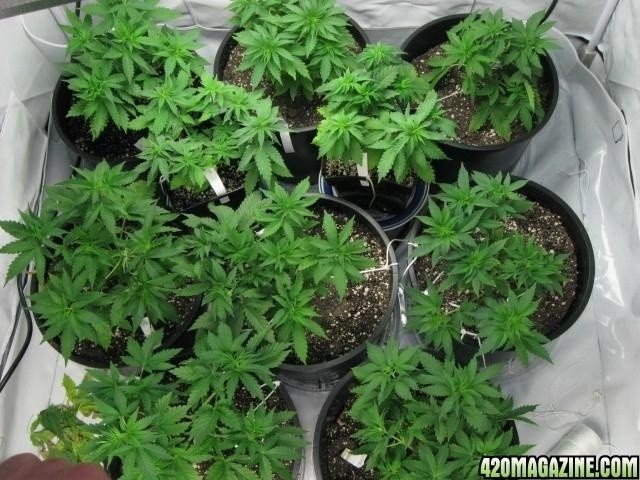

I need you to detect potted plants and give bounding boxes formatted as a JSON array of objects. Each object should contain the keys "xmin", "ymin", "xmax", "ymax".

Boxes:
[
  {"xmin": 52, "ymin": 0, "xmax": 211, "ymax": 165},
  {"xmin": 141, "ymin": 82, "xmax": 293, "ymax": 214},
  {"xmin": 408, "ymin": 162, "xmax": 594, "ymax": 363},
  {"xmin": 312, "ymin": 337, "xmax": 539, "ymax": 480},
  {"xmin": 0, "ymin": 153, "xmax": 205, "ymax": 368},
  {"xmin": 311, "ymin": 39, "xmax": 457, "ymax": 249},
  {"xmin": 396, "ymin": 6, "xmax": 564, "ymax": 172},
  {"xmin": 175, "ymin": 173, "xmax": 399, "ymax": 381},
  {"xmin": 28, "ymin": 322, "xmax": 310, "ymax": 480},
  {"xmin": 212, "ymin": 0, "xmax": 374, "ymax": 182}
]
